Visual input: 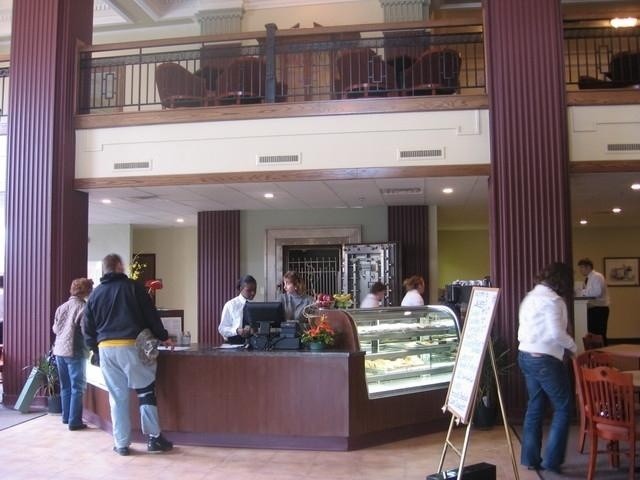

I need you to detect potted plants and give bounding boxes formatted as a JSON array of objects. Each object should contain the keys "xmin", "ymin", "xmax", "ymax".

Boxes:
[
  {"xmin": 38, "ymin": 359, "xmax": 61, "ymax": 414},
  {"xmin": 473, "ymin": 336, "xmax": 516, "ymax": 431}
]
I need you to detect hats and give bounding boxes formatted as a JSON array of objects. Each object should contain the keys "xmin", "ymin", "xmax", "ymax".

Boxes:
[
  {"xmin": 133, "ymin": 328, "xmax": 160, "ymax": 368},
  {"xmin": 69, "ymin": 278, "xmax": 94, "ymax": 298}
]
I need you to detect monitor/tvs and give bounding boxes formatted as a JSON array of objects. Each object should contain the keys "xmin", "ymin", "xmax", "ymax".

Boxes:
[{"xmin": 245, "ymin": 301, "xmax": 286, "ymax": 338}]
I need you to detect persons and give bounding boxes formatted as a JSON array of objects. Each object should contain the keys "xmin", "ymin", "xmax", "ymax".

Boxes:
[
  {"xmin": 84, "ymin": 254, "xmax": 173, "ymax": 454},
  {"xmin": 52, "ymin": 277, "xmax": 93, "ymax": 432},
  {"xmin": 217, "ymin": 275, "xmax": 257, "ymax": 344},
  {"xmin": 275, "ymin": 269, "xmax": 316, "ymax": 322},
  {"xmin": 517, "ymin": 264, "xmax": 579, "ymax": 476},
  {"xmin": 576, "ymin": 259, "xmax": 610, "ymax": 349},
  {"xmin": 401, "ymin": 276, "xmax": 425, "ymax": 308},
  {"xmin": 360, "ymin": 281, "xmax": 385, "ymax": 309}
]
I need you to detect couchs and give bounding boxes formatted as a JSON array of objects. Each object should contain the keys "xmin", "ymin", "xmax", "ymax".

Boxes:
[
  {"xmin": 401, "ymin": 49, "xmax": 462, "ymax": 94},
  {"xmin": 207, "ymin": 54, "xmax": 262, "ymax": 105},
  {"xmin": 336, "ymin": 47, "xmax": 394, "ymax": 97},
  {"xmin": 154, "ymin": 63, "xmax": 208, "ymax": 110}
]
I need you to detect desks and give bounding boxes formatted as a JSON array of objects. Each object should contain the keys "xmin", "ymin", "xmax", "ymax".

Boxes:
[
  {"xmin": 612, "ymin": 370, "xmax": 639, "ymax": 468},
  {"xmin": 607, "ymin": 368, "xmax": 638, "ymax": 387},
  {"xmin": 597, "ymin": 342, "xmax": 640, "ymax": 363}
]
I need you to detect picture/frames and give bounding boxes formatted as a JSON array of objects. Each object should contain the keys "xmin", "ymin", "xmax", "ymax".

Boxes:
[{"xmin": 604, "ymin": 257, "xmax": 639, "ymax": 288}]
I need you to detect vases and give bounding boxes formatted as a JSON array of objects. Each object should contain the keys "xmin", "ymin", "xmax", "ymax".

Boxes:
[{"xmin": 308, "ymin": 342, "xmax": 325, "ymax": 350}]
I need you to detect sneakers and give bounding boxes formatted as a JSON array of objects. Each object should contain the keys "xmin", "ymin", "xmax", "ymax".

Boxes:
[
  {"xmin": 69, "ymin": 423, "xmax": 88, "ymax": 431},
  {"xmin": 112, "ymin": 445, "xmax": 129, "ymax": 455},
  {"xmin": 147, "ymin": 433, "xmax": 174, "ymax": 452}
]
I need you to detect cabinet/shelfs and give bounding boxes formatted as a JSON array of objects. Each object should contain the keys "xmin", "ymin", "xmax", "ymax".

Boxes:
[{"xmin": 321, "ymin": 305, "xmax": 461, "ymax": 400}]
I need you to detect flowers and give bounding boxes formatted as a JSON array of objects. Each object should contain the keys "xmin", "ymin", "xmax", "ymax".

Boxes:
[
  {"xmin": 127, "ymin": 249, "xmax": 148, "ymax": 279},
  {"xmin": 301, "ymin": 314, "xmax": 336, "ymax": 346}
]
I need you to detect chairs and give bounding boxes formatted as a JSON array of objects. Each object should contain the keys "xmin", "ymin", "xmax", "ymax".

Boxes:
[
  {"xmin": 571, "ymin": 353, "xmax": 597, "ymax": 454},
  {"xmin": 582, "ymin": 333, "xmax": 604, "ymax": 367},
  {"xmin": 579, "ymin": 365, "xmax": 639, "ymax": 480}
]
